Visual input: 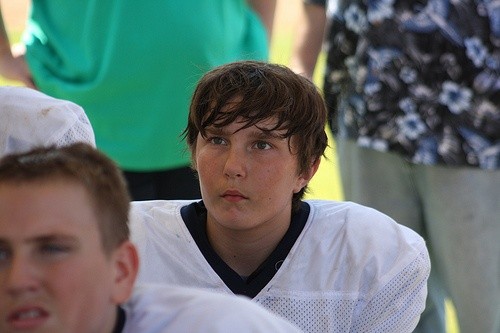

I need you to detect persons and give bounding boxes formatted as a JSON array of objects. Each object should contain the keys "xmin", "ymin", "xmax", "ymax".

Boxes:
[
  {"xmin": 0, "ymin": 0, "xmax": 276, "ymax": 201},
  {"xmin": 292, "ymin": 0, "xmax": 500, "ymax": 333},
  {"xmin": 0, "ymin": 62, "xmax": 433, "ymax": 333}
]
